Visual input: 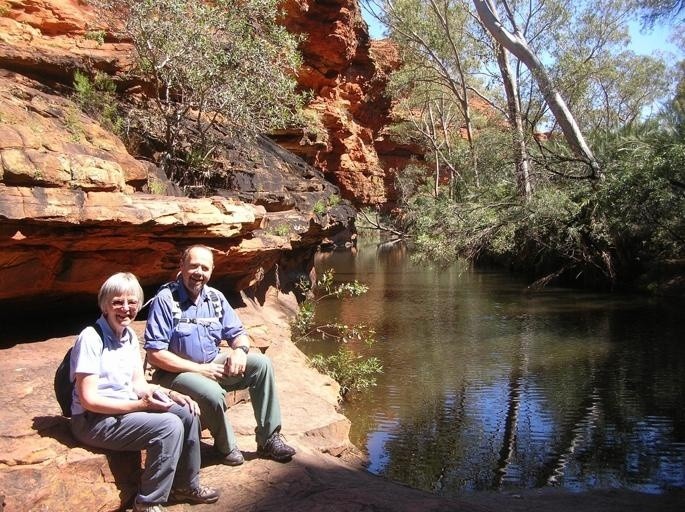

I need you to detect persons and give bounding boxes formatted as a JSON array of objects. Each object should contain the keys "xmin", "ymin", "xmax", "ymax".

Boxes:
[
  {"xmin": 142, "ymin": 242, "xmax": 296, "ymax": 465},
  {"xmin": 52, "ymin": 269, "xmax": 220, "ymax": 512}
]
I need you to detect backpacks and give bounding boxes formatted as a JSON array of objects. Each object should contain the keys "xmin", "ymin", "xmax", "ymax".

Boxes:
[{"xmin": 54, "ymin": 323, "xmax": 104, "ymax": 416}]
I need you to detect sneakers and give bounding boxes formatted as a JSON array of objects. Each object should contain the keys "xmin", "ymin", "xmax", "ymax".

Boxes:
[
  {"xmin": 133, "ymin": 496, "xmax": 168, "ymax": 512},
  {"xmin": 257, "ymin": 427, "xmax": 296, "ymax": 460},
  {"xmin": 169, "ymin": 484, "xmax": 221, "ymax": 503},
  {"xmin": 214, "ymin": 444, "xmax": 245, "ymax": 465}
]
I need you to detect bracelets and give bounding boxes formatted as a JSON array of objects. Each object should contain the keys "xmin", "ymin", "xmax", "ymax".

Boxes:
[
  {"xmin": 168, "ymin": 389, "xmax": 174, "ymax": 396},
  {"xmin": 236, "ymin": 344, "xmax": 250, "ymax": 355}
]
